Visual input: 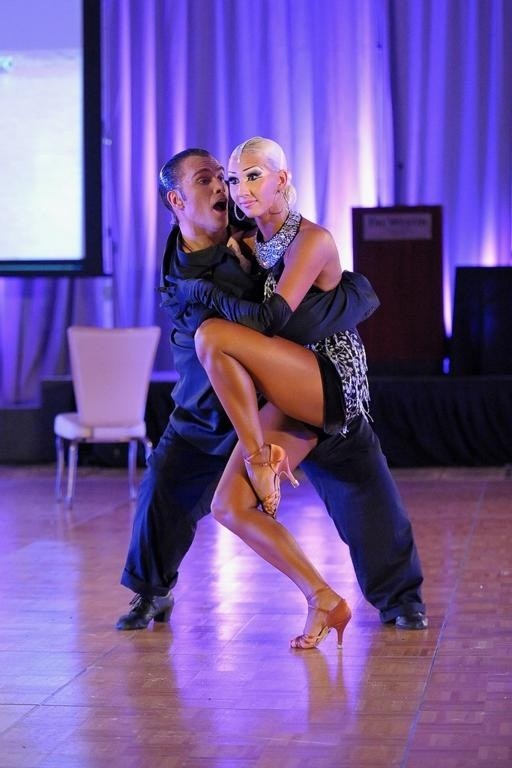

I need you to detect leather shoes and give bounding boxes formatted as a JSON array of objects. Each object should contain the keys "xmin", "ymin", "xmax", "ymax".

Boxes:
[{"xmin": 395, "ymin": 610, "xmax": 429, "ymax": 630}]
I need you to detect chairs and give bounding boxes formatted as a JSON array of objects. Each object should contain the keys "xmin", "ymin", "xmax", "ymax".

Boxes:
[{"xmin": 51, "ymin": 324, "xmax": 164, "ymax": 511}]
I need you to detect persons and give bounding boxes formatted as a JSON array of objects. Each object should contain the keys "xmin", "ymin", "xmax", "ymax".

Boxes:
[
  {"xmin": 105, "ymin": 144, "xmax": 431, "ymax": 634},
  {"xmin": 156, "ymin": 132, "xmax": 371, "ymax": 652}
]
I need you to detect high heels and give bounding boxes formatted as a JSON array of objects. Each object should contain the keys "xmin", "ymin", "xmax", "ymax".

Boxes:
[
  {"xmin": 115, "ymin": 590, "xmax": 175, "ymax": 631},
  {"xmin": 289, "ymin": 585, "xmax": 352, "ymax": 650},
  {"xmin": 244, "ymin": 444, "xmax": 300, "ymax": 518}
]
